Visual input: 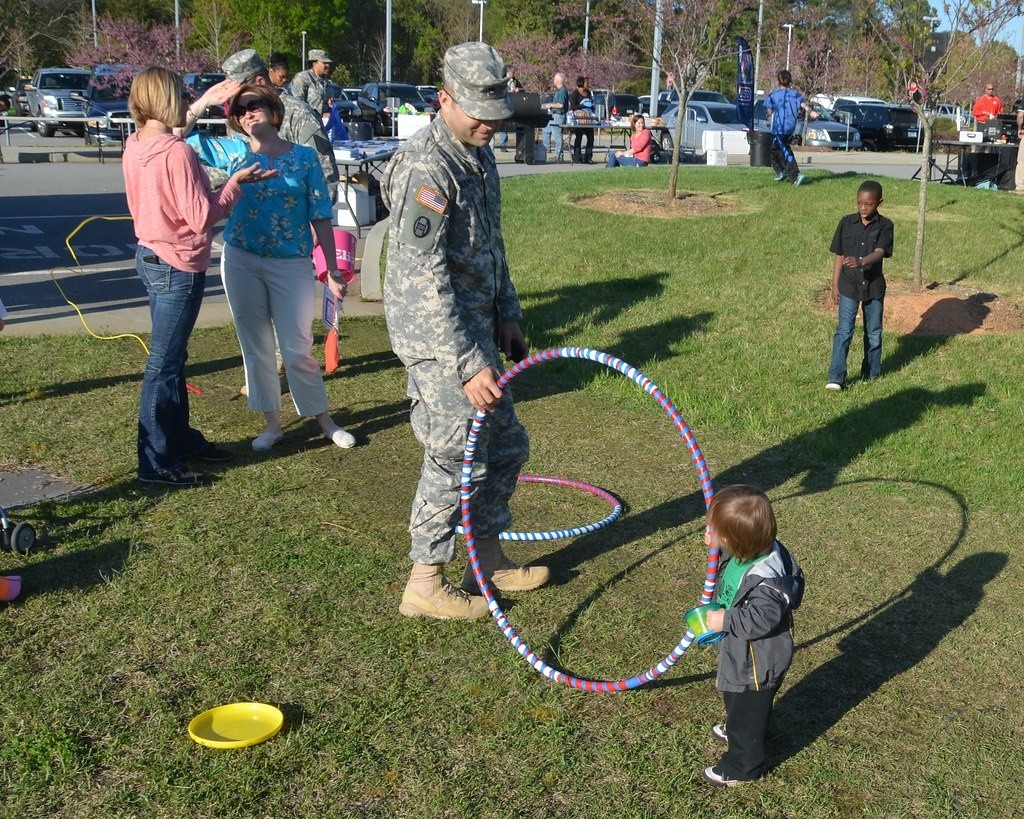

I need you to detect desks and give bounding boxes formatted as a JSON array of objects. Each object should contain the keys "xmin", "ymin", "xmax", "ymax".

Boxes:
[
  {"xmin": 932, "ymin": 140, "xmax": 1020, "ymax": 187},
  {"xmin": 558, "ymin": 124, "xmax": 675, "ymax": 166},
  {"xmin": 333, "ymin": 147, "xmax": 406, "ymax": 239}
]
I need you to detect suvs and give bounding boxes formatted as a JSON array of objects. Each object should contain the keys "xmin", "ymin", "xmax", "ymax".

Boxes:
[
  {"xmin": 357, "ymin": 82, "xmax": 437, "ymax": 137},
  {"xmin": 831, "ymin": 104, "xmax": 924, "ymax": 153},
  {"xmin": 753, "ymin": 99, "xmax": 863, "ymax": 149},
  {"xmin": 25, "ymin": 67, "xmax": 91, "ymax": 138},
  {"xmin": 70, "ymin": 63, "xmax": 145, "ymax": 147},
  {"xmin": 183, "ymin": 73, "xmax": 226, "ymax": 104},
  {"xmin": 592, "ymin": 95, "xmax": 642, "ymax": 119}
]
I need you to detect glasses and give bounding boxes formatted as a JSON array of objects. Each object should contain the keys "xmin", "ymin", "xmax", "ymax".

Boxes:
[
  {"xmin": 235, "ymin": 99, "xmax": 264, "ymax": 119},
  {"xmin": 987, "ymin": 88, "xmax": 994, "ymax": 90}
]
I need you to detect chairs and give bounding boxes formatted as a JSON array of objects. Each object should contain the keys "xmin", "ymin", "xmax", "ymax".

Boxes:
[
  {"xmin": 46, "ymin": 75, "xmax": 60, "ymax": 86},
  {"xmin": 854, "ymin": 112, "xmax": 863, "ymax": 120},
  {"xmin": 64, "ymin": 78, "xmax": 77, "ymax": 85},
  {"xmin": 380, "ymin": 92, "xmax": 385, "ymax": 101},
  {"xmin": 97, "ymin": 86, "xmax": 116, "ymax": 100}
]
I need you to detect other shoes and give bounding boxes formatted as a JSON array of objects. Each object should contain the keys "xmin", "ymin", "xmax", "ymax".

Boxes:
[
  {"xmin": 322, "ymin": 423, "xmax": 356, "ymax": 449},
  {"xmin": 703, "ymin": 765, "xmax": 738, "ymax": 788},
  {"xmin": 251, "ymin": 428, "xmax": 283, "ymax": 451},
  {"xmin": 1008, "ymin": 189, "xmax": 1024, "ymax": 194},
  {"xmin": 796, "ymin": 172, "xmax": 806, "ymax": 186},
  {"xmin": 712, "ymin": 723, "xmax": 728, "ymax": 743},
  {"xmin": 501, "ymin": 148, "xmax": 508, "ymax": 152},
  {"xmin": 773, "ymin": 170, "xmax": 787, "ymax": 181},
  {"xmin": 585, "ymin": 159, "xmax": 594, "ymax": 164},
  {"xmin": 826, "ymin": 381, "xmax": 845, "ymax": 392},
  {"xmin": 138, "ymin": 443, "xmax": 233, "ymax": 484}
]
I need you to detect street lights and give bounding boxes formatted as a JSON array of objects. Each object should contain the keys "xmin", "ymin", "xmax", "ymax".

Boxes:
[
  {"xmin": 472, "ymin": 0, "xmax": 486, "ymax": 43},
  {"xmin": 783, "ymin": 24, "xmax": 794, "ymax": 71},
  {"xmin": 301, "ymin": 31, "xmax": 307, "ymax": 71},
  {"xmin": 922, "ymin": 16, "xmax": 940, "ymax": 33}
]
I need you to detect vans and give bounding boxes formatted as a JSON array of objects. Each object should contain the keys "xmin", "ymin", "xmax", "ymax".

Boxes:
[{"xmin": 832, "ymin": 97, "xmax": 887, "ymax": 125}]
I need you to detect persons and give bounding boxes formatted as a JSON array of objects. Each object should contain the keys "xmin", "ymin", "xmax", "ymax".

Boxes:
[
  {"xmin": 500, "ymin": 65, "xmax": 525, "ymax": 152},
  {"xmin": 385, "ymin": 41, "xmax": 551, "ymax": 625},
  {"xmin": 704, "ymin": 484, "xmax": 805, "ymax": 787},
  {"xmin": 973, "ymin": 83, "xmax": 1024, "ymax": 194},
  {"xmin": 543, "ymin": 73, "xmax": 597, "ymax": 165},
  {"xmin": 605, "ymin": 115, "xmax": 651, "ymax": 168},
  {"xmin": 123, "ymin": 46, "xmax": 356, "ymax": 488},
  {"xmin": 825, "ymin": 181, "xmax": 894, "ymax": 391},
  {"xmin": 762, "ymin": 70, "xmax": 820, "ymax": 189}
]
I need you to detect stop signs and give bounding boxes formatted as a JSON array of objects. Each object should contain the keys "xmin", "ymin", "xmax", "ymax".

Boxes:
[{"xmin": 910, "ymin": 81, "xmax": 917, "ymax": 93}]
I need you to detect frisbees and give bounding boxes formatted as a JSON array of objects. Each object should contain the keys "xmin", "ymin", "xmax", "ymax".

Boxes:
[{"xmin": 188, "ymin": 702, "xmax": 284, "ymax": 750}]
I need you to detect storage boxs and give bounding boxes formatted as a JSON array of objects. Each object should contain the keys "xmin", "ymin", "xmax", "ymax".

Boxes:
[
  {"xmin": 331, "ymin": 170, "xmax": 390, "ymax": 227},
  {"xmin": 398, "ymin": 114, "xmax": 435, "ymax": 137},
  {"xmin": 701, "ymin": 130, "xmax": 751, "ymax": 167},
  {"xmin": 959, "ymin": 131, "xmax": 984, "ymax": 143}
]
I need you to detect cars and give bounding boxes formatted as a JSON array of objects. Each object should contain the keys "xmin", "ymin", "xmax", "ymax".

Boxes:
[
  {"xmin": 639, "ymin": 90, "xmax": 731, "ymax": 117},
  {"xmin": 417, "ymin": 85, "xmax": 440, "ymax": 109},
  {"xmin": 333, "ymin": 90, "xmax": 373, "ymax": 142},
  {"xmin": 923, "ymin": 104, "xmax": 974, "ymax": 124},
  {"xmin": 9, "ymin": 79, "xmax": 32, "ymax": 117},
  {"xmin": 659, "ymin": 100, "xmax": 749, "ymax": 152},
  {"xmin": 343, "ymin": 88, "xmax": 365, "ymax": 106}
]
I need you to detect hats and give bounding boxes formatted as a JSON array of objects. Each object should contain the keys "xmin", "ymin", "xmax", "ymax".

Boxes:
[
  {"xmin": 308, "ymin": 49, "xmax": 333, "ymax": 63},
  {"xmin": 221, "ymin": 49, "xmax": 265, "ymax": 84},
  {"xmin": 444, "ymin": 42, "xmax": 514, "ymax": 120}
]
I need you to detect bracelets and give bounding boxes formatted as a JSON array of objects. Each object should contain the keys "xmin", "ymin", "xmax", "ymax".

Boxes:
[
  {"xmin": 188, "ymin": 108, "xmax": 199, "ymax": 120},
  {"xmin": 808, "ymin": 109, "xmax": 812, "ymax": 114}
]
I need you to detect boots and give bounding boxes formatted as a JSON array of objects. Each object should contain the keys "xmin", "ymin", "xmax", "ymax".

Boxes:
[
  {"xmin": 461, "ymin": 534, "xmax": 550, "ymax": 592},
  {"xmin": 399, "ymin": 562, "xmax": 489, "ymax": 621}
]
